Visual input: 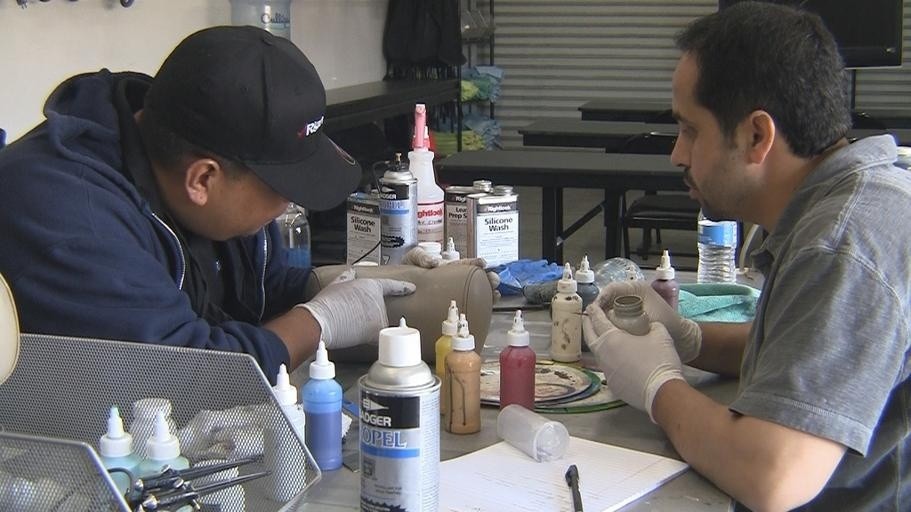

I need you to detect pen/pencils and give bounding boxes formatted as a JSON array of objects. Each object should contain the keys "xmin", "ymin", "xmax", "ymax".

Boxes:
[{"xmin": 564, "ymin": 464, "xmax": 583, "ymax": 512}]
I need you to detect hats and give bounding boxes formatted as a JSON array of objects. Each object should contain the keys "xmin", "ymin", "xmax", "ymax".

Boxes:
[{"xmin": 150, "ymin": 27, "xmax": 362, "ymax": 212}]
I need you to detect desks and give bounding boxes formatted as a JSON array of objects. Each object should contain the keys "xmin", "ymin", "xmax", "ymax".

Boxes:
[{"xmin": 265, "ymin": 80, "xmax": 744, "ymax": 512}]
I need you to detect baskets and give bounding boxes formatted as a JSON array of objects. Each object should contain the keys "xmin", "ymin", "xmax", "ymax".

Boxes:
[{"xmin": 0, "ymin": 335, "xmax": 323, "ymax": 512}]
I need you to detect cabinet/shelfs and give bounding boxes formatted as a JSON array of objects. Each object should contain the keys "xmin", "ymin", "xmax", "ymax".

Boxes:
[{"xmin": 397, "ymin": 1, "xmax": 497, "ymax": 154}]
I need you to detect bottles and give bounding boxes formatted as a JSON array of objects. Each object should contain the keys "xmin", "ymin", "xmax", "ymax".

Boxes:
[
  {"xmin": 697, "ymin": 212, "xmax": 735, "ymax": 280},
  {"xmin": 380, "ymin": 150, "xmax": 417, "ymax": 267},
  {"xmin": 305, "ymin": 342, "xmax": 345, "ymax": 474},
  {"xmin": 444, "ymin": 179, "xmax": 491, "ymax": 267},
  {"xmin": 499, "ymin": 309, "xmax": 536, "ymax": 412},
  {"xmin": 129, "ymin": 397, "xmax": 176, "ymax": 457},
  {"xmin": 443, "ymin": 311, "xmax": 480, "ymax": 431},
  {"xmin": 262, "ymin": 366, "xmax": 311, "ymax": 501},
  {"xmin": 138, "ymin": 413, "xmax": 191, "ymax": 512},
  {"xmin": 548, "ymin": 263, "xmax": 583, "ymax": 363},
  {"xmin": 608, "ymin": 293, "xmax": 649, "ymax": 336},
  {"xmin": 347, "ymin": 191, "xmax": 381, "ymax": 266},
  {"xmin": 577, "ymin": 251, "xmax": 601, "ymax": 308},
  {"xmin": 468, "ymin": 186, "xmax": 526, "ymax": 270},
  {"xmin": 99, "ymin": 406, "xmax": 137, "ymax": 510},
  {"xmin": 653, "ymin": 248, "xmax": 680, "ymax": 314},
  {"xmin": 444, "ymin": 238, "xmax": 463, "ymax": 262},
  {"xmin": 418, "ymin": 240, "xmax": 443, "ymax": 261},
  {"xmin": 190, "ymin": 461, "xmax": 247, "ymax": 510},
  {"xmin": 435, "ymin": 300, "xmax": 459, "ymax": 369},
  {"xmin": 271, "ymin": 197, "xmax": 310, "ymax": 271},
  {"xmin": 356, "ymin": 319, "xmax": 442, "ymax": 512}
]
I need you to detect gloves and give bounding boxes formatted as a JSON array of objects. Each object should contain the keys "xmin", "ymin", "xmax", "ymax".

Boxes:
[
  {"xmin": 580, "ymin": 280, "xmax": 702, "ymax": 364},
  {"xmin": 402, "ymin": 247, "xmax": 501, "ymax": 304},
  {"xmin": 485, "ymin": 258, "xmax": 565, "ymax": 294},
  {"xmin": 294, "ymin": 268, "xmax": 417, "ymax": 351},
  {"xmin": 581, "ymin": 305, "xmax": 687, "ymax": 425}
]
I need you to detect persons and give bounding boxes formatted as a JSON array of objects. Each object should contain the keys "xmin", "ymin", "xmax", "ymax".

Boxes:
[
  {"xmin": 0, "ymin": 25, "xmax": 503, "ymax": 442},
  {"xmin": 580, "ymin": 0, "xmax": 911, "ymax": 512}
]
